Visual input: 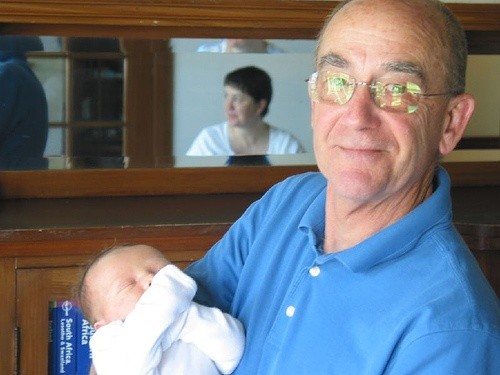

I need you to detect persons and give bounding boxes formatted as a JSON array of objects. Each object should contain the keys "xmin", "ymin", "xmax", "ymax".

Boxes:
[
  {"xmin": 78, "ymin": 244, "xmax": 245, "ymax": 375},
  {"xmin": 90, "ymin": 0, "xmax": 499, "ymax": 375},
  {"xmin": 183, "ymin": 66, "xmax": 305, "ymax": 155},
  {"xmin": 1, "ymin": 37, "xmax": 48, "ymax": 157}
]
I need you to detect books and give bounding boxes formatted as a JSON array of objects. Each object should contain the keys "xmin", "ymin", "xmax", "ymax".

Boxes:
[{"xmin": 49, "ymin": 302, "xmax": 96, "ymax": 375}]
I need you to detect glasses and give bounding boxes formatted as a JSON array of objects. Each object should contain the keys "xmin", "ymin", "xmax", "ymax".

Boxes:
[{"xmin": 305, "ymin": 70, "xmax": 455, "ymax": 115}]
